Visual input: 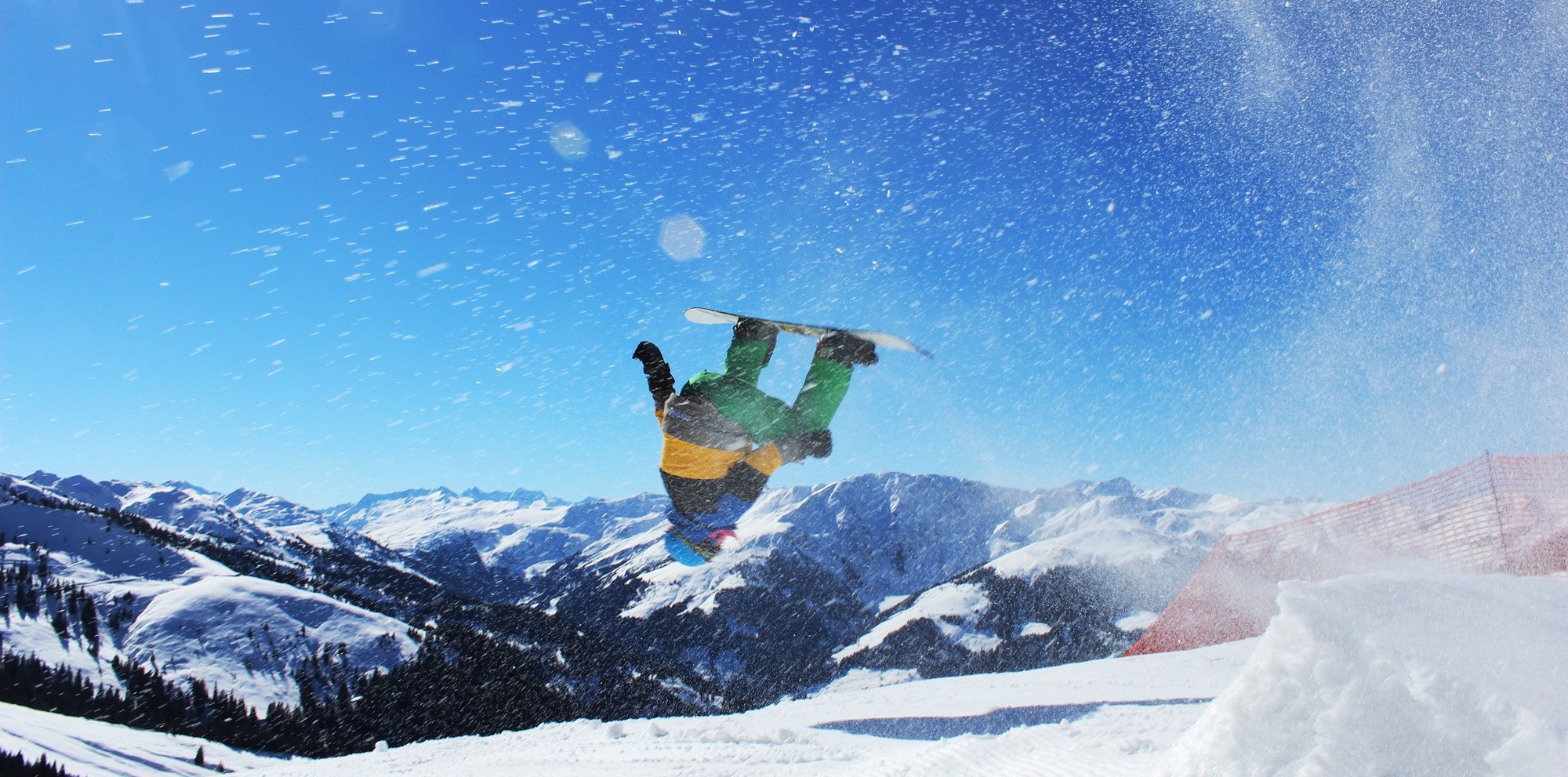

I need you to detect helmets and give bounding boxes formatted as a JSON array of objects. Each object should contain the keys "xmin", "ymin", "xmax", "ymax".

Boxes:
[{"xmin": 665, "ymin": 526, "xmax": 719, "ymax": 567}]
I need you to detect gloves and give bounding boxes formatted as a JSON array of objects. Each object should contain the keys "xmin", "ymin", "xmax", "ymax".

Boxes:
[
  {"xmin": 803, "ymin": 429, "xmax": 832, "ymax": 459},
  {"xmin": 632, "ymin": 342, "xmax": 666, "ymax": 374}
]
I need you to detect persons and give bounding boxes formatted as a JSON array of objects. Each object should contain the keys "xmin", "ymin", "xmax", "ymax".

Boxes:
[{"xmin": 632, "ymin": 319, "xmax": 879, "ymax": 567}]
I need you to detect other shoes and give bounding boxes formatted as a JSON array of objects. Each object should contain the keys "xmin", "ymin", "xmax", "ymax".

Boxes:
[
  {"xmin": 815, "ymin": 332, "xmax": 879, "ymax": 368},
  {"xmin": 732, "ymin": 318, "xmax": 776, "ymax": 369}
]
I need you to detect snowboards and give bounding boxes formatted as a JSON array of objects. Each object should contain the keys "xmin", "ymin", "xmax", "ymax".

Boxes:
[{"xmin": 683, "ymin": 305, "xmax": 935, "ymax": 370}]
